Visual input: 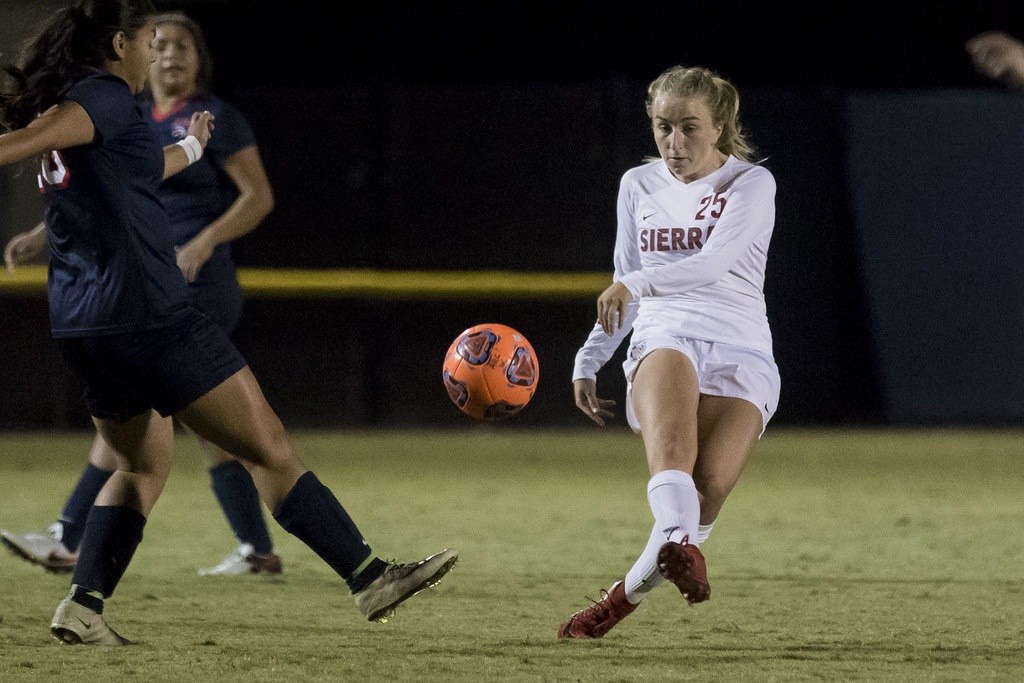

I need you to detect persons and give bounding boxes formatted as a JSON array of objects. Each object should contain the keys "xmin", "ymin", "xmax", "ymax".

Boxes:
[
  {"xmin": 0, "ymin": 1, "xmax": 460, "ymax": 649},
  {"xmin": 556, "ymin": 64, "xmax": 783, "ymax": 640}
]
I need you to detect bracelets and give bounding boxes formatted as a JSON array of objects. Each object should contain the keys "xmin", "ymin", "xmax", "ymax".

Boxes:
[{"xmin": 177, "ymin": 135, "xmax": 202, "ymax": 166}]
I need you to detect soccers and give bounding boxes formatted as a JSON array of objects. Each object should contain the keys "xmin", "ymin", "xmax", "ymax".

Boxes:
[{"xmin": 441, "ymin": 321, "xmax": 539, "ymax": 422}]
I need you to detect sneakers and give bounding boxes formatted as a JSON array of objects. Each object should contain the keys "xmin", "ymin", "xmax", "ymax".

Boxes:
[
  {"xmin": 558, "ymin": 579, "xmax": 642, "ymax": 639},
  {"xmin": 198, "ymin": 543, "xmax": 281, "ymax": 577},
  {"xmin": 49, "ymin": 599, "xmax": 131, "ymax": 646},
  {"xmin": 657, "ymin": 535, "xmax": 711, "ymax": 603},
  {"xmin": 0, "ymin": 522, "xmax": 81, "ymax": 574},
  {"xmin": 342, "ymin": 549, "xmax": 458, "ymax": 622}
]
